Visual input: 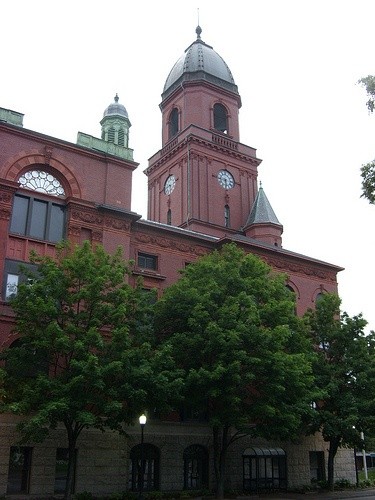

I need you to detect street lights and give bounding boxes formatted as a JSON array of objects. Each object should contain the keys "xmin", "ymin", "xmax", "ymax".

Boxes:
[
  {"xmin": 352, "ymin": 426, "xmax": 358, "ymax": 490},
  {"xmin": 138, "ymin": 414, "xmax": 147, "ymax": 500}
]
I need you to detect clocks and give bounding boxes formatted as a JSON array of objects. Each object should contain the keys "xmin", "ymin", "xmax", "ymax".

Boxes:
[
  {"xmin": 218, "ymin": 169, "xmax": 235, "ymax": 189},
  {"xmin": 165, "ymin": 174, "xmax": 176, "ymax": 195}
]
